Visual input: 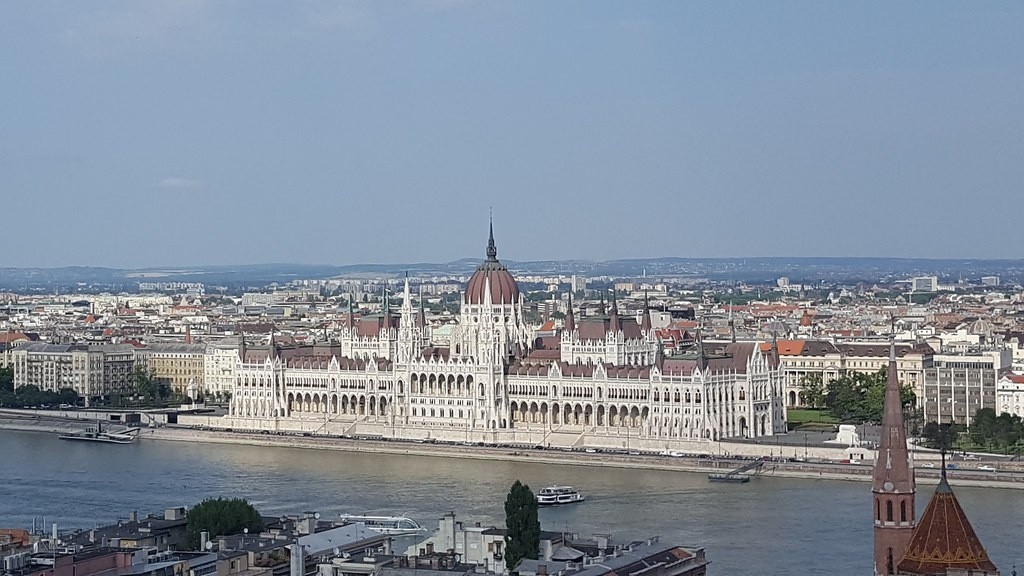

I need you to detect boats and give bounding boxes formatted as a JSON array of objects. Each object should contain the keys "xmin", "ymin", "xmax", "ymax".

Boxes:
[
  {"xmin": 708, "ymin": 472, "xmax": 749, "ymax": 483},
  {"xmin": 58, "ymin": 420, "xmax": 132, "ymax": 445},
  {"xmin": 339, "ymin": 512, "xmax": 428, "ymax": 537},
  {"xmin": 537, "ymin": 485, "xmax": 584, "ymax": 505}
]
